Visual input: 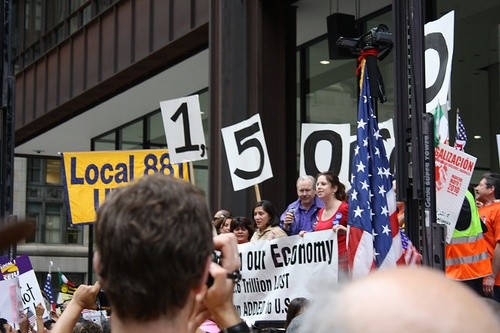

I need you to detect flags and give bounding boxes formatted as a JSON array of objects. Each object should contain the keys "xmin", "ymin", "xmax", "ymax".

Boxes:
[
  {"xmin": 346, "ymin": 58, "xmax": 421, "ymax": 278},
  {"xmin": 455, "ymin": 116, "xmax": 467, "ymax": 150},
  {"xmin": 44, "ymin": 273, "xmax": 57, "ymax": 312},
  {"xmin": 56, "ymin": 272, "xmax": 78, "ymax": 305},
  {"xmin": 435, "ymin": 106, "xmax": 449, "ymax": 145},
  {"xmin": 26, "ymin": 311, "xmax": 36, "ymax": 324}
]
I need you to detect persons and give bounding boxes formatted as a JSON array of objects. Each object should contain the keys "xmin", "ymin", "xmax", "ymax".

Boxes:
[
  {"xmin": 251, "ymin": 200, "xmax": 289, "ymax": 244},
  {"xmin": 0, "ymin": 173, "xmax": 254, "ymax": 333},
  {"xmin": 280, "ymin": 173, "xmax": 500, "ymax": 333}
]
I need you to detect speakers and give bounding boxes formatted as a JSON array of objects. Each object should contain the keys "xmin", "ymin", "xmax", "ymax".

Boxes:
[{"xmin": 327, "ymin": 13, "xmax": 357, "ymax": 60}]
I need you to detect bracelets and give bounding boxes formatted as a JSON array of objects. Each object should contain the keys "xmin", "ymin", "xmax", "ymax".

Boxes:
[{"xmin": 220, "ymin": 321, "xmax": 251, "ymax": 333}]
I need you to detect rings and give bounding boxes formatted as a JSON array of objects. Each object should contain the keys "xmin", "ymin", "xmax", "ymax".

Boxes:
[{"xmin": 489, "ymin": 289, "xmax": 490, "ymax": 291}]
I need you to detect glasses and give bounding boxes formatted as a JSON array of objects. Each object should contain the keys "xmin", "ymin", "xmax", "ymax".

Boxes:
[{"xmin": 214, "ymin": 217, "xmax": 220, "ymax": 221}]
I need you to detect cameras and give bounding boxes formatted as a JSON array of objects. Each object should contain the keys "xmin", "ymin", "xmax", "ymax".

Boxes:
[
  {"xmin": 96, "ymin": 290, "xmax": 111, "ymax": 310},
  {"xmin": 207, "ymin": 253, "xmax": 221, "ymax": 288}
]
